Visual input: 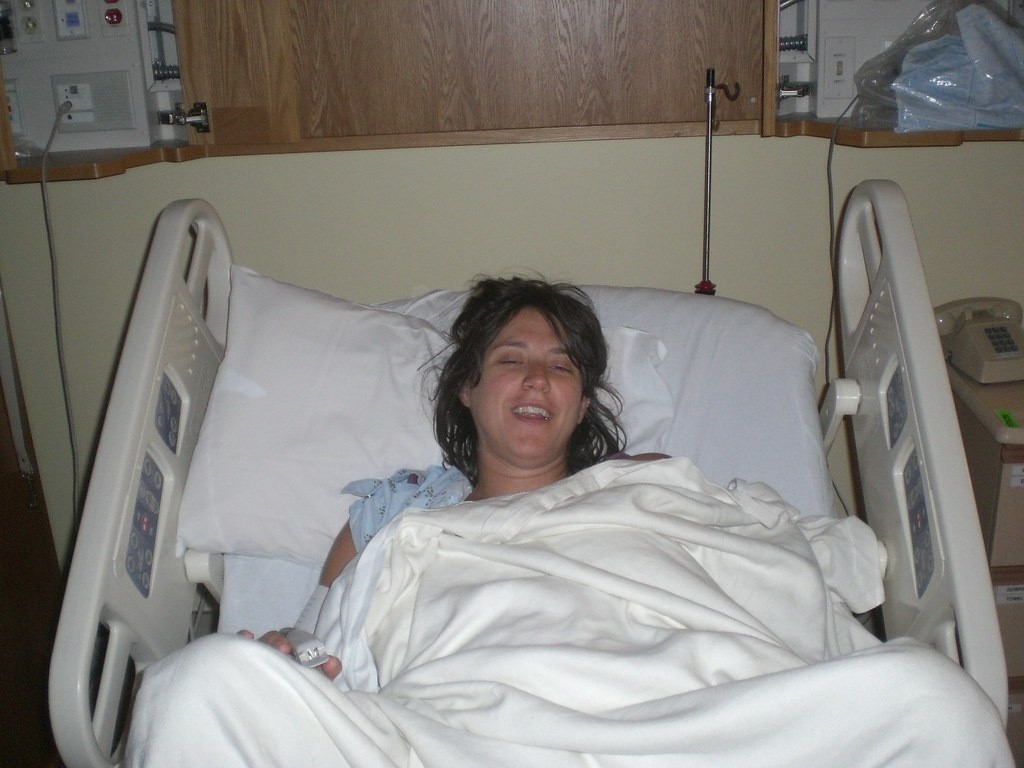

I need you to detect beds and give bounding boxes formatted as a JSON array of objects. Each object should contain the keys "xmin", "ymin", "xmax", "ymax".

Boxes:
[{"xmin": 46, "ymin": 177, "xmax": 1024, "ymax": 768}]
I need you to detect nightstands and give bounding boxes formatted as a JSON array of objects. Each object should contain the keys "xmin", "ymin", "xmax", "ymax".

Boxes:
[{"xmin": 946, "ymin": 358, "xmax": 1024, "ymax": 756}]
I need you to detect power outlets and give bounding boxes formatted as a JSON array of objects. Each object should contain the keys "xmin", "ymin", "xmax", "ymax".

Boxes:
[
  {"xmin": 98, "ymin": 1, "xmax": 131, "ymax": 38},
  {"xmin": 16, "ymin": 0, "xmax": 45, "ymax": 45},
  {"xmin": 51, "ymin": 68, "xmax": 137, "ymax": 134},
  {"xmin": 51, "ymin": 0, "xmax": 91, "ymax": 42}
]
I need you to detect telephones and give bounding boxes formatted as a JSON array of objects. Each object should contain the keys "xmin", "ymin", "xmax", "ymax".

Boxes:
[{"xmin": 932, "ymin": 296, "xmax": 1024, "ymax": 384}]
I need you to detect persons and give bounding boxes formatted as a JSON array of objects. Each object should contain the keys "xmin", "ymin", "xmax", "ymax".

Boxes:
[{"xmin": 122, "ymin": 277, "xmax": 1014, "ymax": 768}]
